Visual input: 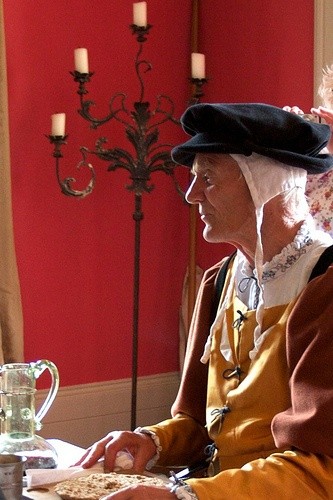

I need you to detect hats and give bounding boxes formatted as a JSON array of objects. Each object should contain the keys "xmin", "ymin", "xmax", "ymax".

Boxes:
[{"xmin": 171, "ymin": 103, "xmax": 333, "ymax": 174}]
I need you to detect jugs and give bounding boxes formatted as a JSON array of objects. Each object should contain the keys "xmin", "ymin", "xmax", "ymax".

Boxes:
[{"xmin": 0, "ymin": 357, "xmax": 61, "ymax": 482}]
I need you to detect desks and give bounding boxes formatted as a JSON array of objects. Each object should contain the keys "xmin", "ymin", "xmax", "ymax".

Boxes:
[{"xmin": 0, "ymin": 435, "xmax": 176, "ymax": 500}]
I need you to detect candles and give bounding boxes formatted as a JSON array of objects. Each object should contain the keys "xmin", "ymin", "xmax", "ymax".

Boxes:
[
  {"xmin": 51, "ymin": 113, "xmax": 66, "ymax": 136},
  {"xmin": 73, "ymin": 47, "xmax": 89, "ymax": 73},
  {"xmin": 191, "ymin": 53, "xmax": 206, "ymax": 78},
  {"xmin": 132, "ymin": 1, "xmax": 147, "ymax": 26}
]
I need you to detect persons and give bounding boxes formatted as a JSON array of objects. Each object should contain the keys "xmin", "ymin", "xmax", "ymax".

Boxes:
[
  {"xmin": 282, "ymin": 63, "xmax": 333, "ymax": 239},
  {"xmin": 69, "ymin": 102, "xmax": 333, "ymax": 500}
]
